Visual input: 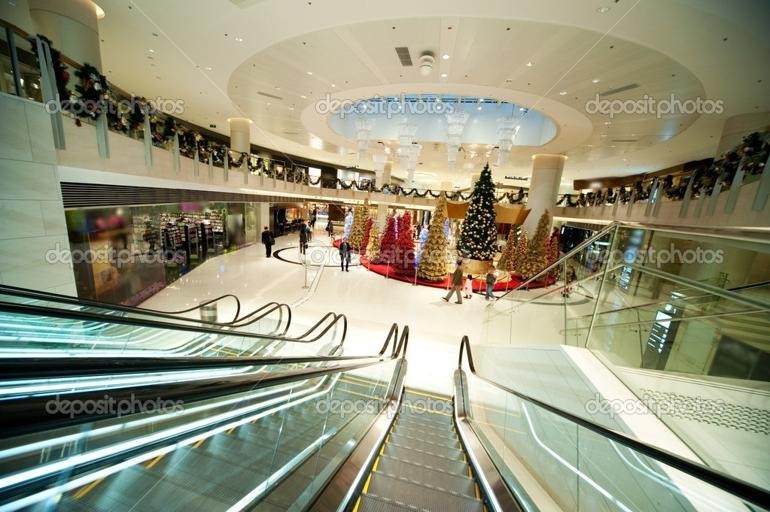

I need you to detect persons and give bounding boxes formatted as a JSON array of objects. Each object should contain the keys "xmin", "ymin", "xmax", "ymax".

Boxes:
[
  {"xmin": 484, "ymin": 264, "xmax": 496, "ymax": 301},
  {"xmin": 304, "ymin": 222, "xmax": 313, "ymax": 241},
  {"xmin": 339, "ymin": 236, "xmax": 351, "ymax": 272},
  {"xmin": 309, "ymin": 208, "xmax": 317, "ymax": 228},
  {"xmin": 462, "ymin": 274, "xmax": 473, "ymax": 299},
  {"xmin": 300, "ymin": 229, "xmax": 307, "ymax": 255},
  {"xmin": 299, "ymin": 220, "xmax": 306, "ymax": 233},
  {"xmin": 261, "ymin": 226, "xmax": 274, "ymax": 257},
  {"xmin": 561, "ymin": 259, "xmax": 576, "ymax": 297},
  {"xmin": 441, "ymin": 259, "xmax": 463, "ymax": 304},
  {"xmin": 326, "ymin": 218, "xmax": 333, "ymax": 237}
]
